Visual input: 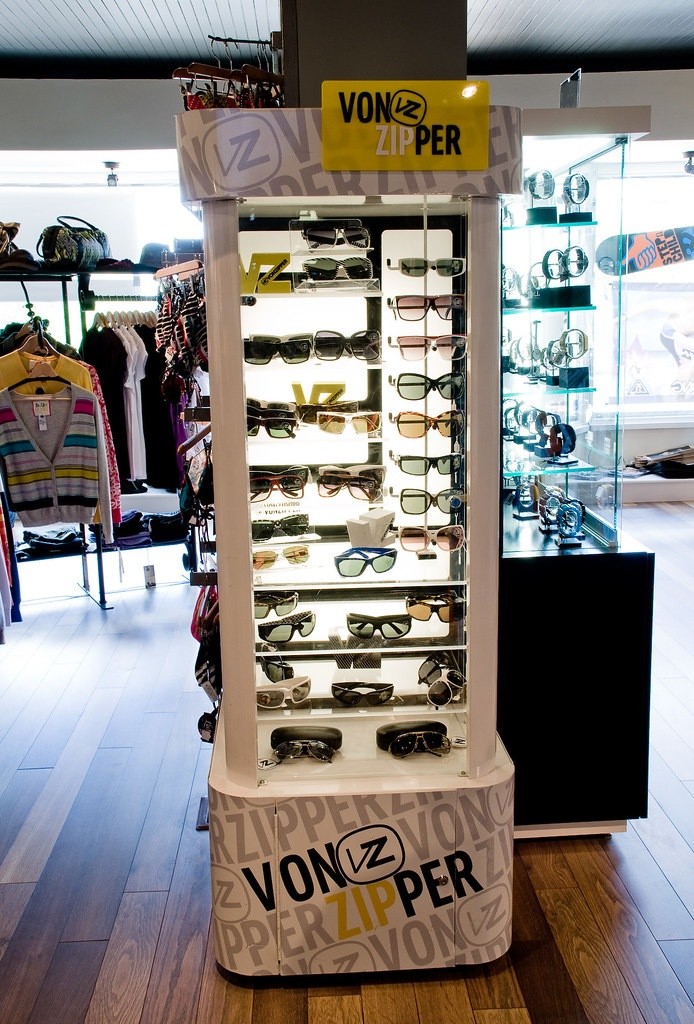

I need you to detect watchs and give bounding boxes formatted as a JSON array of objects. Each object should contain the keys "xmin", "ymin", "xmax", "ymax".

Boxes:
[
  {"xmin": 501, "ymin": 326, "xmax": 589, "ymax": 373},
  {"xmin": 514, "ymin": 483, "xmax": 585, "ymax": 536},
  {"xmin": 502, "ymin": 168, "xmax": 589, "ymax": 226},
  {"xmin": 502, "ymin": 398, "xmax": 577, "ymax": 455},
  {"xmin": 502, "ymin": 246, "xmax": 589, "ymax": 298}
]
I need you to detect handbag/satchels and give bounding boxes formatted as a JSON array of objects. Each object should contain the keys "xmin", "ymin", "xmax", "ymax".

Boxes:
[{"xmin": 35, "ymin": 216, "xmax": 112, "ymax": 266}]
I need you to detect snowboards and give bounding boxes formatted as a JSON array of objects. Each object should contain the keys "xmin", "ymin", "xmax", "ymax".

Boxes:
[{"xmin": 595, "ymin": 225, "xmax": 694, "ymax": 276}]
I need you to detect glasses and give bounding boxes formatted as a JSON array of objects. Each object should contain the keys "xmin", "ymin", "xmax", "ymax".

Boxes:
[
  {"xmin": 273, "ymin": 740, "xmax": 336, "ymax": 763},
  {"xmin": 390, "ymin": 294, "xmax": 465, "ymax": 322},
  {"xmin": 301, "ymin": 227, "xmax": 371, "ymax": 254},
  {"xmin": 302, "ymin": 257, "xmax": 374, "ymax": 286},
  {"xmin": 388, "ymin": 257, "xmax": 468, "ymax": 278},
  {"xmin": 245, "ymin": 329, "xmax": 468, "ymax": 711},
  {"xmin": 388, "ymin": 731, "xmax": 452, "ymax": 759}
]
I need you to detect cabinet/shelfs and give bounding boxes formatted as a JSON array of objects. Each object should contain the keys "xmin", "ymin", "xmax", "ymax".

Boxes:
[
  {"xmin": 492, "ymin": 129, "xmax": 639, "ymax": 554},
  {"xmin": 219, "ymin": 184, "xmax": 505, "ymax": 787}
]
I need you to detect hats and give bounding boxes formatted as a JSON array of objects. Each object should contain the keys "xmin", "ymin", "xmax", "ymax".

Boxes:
[{"xmin": 139, "ymin": 243, "xmax": 174, "ymax": 268}]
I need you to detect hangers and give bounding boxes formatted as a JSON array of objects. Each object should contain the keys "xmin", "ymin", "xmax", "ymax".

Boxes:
[
  {"xmin": 9, "ymin": 303, "xmax": 73, "ymax": 401},
  {"xmin": 92, "ymin": 252, "xmax": 206, "ymax": 330},
  {"xmin": 171, "ymin": 30, "xmax": 283, "ymax": 109}
]
